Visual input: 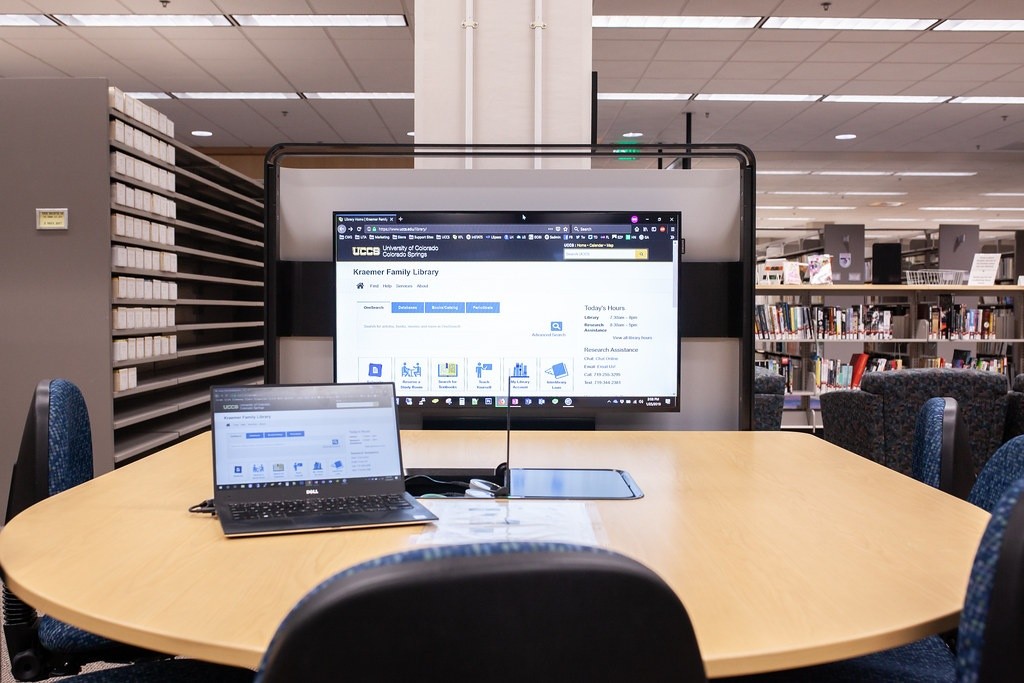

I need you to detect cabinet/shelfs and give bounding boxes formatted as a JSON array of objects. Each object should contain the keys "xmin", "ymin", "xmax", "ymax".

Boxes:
[
  {"xmin": 108, "ymin": 106, "xmax": 267, "ymax": 469},
  {"xmin": 754, "ymin": 221, "xmax": 1024, "ymax": 410}
]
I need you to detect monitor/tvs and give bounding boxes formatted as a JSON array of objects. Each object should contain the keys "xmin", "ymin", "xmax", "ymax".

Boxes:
[{"xmin": 333, "ymin": 211, "xmax": 683, "ymax": 430}]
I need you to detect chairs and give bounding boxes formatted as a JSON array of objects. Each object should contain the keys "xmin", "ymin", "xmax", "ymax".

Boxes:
[
  {"xmin": 820, "ymin": 369, "xmax": 1024, "ymax": 481},
  {"xmin": 769, "ymin": 433, "xmax": 1024, "ymax": 683},
  {"xmin": 913, "ymin": 396, "xmax": 978, "ymax": 501},
  {"xmin": 1, "ymin": 377, "xmax": 177, "ymax": 682},
  {"xmin": 251, "ymin": 540, "xmax": 709, "ymax": 682}
]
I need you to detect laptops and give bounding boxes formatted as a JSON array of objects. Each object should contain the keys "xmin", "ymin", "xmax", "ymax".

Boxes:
[{"xmin": 208, "ymin": 381, "xmax": 440, "ymax": 537}]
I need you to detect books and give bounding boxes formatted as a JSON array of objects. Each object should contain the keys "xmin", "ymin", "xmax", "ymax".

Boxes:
[{"xmin": 753, "ymin": 252, "xmax": 1015, "ymax": 394}]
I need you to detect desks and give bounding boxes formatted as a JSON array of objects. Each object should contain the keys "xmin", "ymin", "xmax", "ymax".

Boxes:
[{"xmin": 0, "ymin": 431, "xmax": 998, "ymax": 678}]
